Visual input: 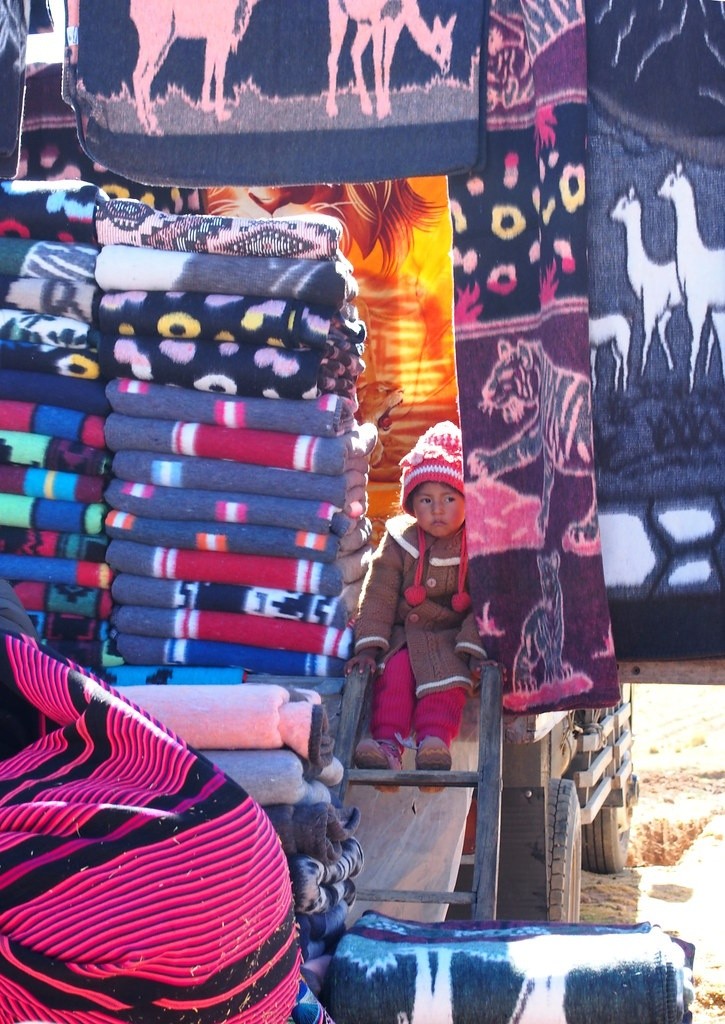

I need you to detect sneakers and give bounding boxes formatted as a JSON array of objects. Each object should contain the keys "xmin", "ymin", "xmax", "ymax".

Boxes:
[
  {"xmin": 359, "ymin": 737, "xmax": 399, "ymax": 785},
  {"xmin": 419, "ymin": 746, "xmax": 454, "ymax": 788}
]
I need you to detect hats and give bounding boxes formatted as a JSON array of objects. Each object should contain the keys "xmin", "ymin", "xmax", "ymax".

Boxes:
[{"xmin": 403, "ymin": 421, "xmax": 475, "ymax": 610}]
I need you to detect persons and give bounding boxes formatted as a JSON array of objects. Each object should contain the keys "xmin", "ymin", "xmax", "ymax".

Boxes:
[{"xmin": 342, "ymin": 420, "xmax": 509, "ymax": 794}]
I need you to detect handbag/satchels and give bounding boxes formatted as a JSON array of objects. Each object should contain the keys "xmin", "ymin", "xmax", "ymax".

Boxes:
[{"xmin": 0, "ymin": 630, "xmax": 299, "ymax": 1024}]
